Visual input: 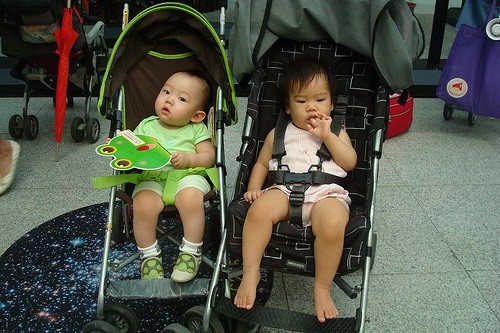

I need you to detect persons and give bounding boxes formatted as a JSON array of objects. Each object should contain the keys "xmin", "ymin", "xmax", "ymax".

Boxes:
[
  {"xmin": 0, "ymin": 138, "xmax": 21, "ymax": 194},
  {"xmin": 233, "ymin": 54, "xmax": 358, "ymax": 322},
  {"xmin": 105, "ymin": 69, "xmax": 219, "ymax": 283}
]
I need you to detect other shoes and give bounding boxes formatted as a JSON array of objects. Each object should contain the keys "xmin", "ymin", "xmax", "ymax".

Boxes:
[
  {"xmin": 171, "ymin": 251, "xmax": 202, "ymax": 282},
  {"xmin": 140, "ymin": 249, "xmax": 164, "ymax": 279},
  {"xmin": 0, "ymin": 139, "xmax": 20, "ymax": 194}
]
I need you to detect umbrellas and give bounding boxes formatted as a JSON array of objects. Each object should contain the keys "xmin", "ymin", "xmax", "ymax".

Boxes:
[{"xmin": 54, "ymin": 0, "xmax": 79, "ymax": 162}]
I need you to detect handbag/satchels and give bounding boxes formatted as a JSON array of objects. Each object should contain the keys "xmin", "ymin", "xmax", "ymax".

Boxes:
[{"xmin": 386, "ymin": 93, "xmax": 413, "ymax": 138}]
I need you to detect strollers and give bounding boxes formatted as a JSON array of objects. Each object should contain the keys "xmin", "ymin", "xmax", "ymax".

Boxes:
[
  {"xmin": 225, "ymin": 0, "xmax": 426, "ymax": 333},
  {"xmin": 82, "ymin": 0, "xmax": 235, "ymax": 333},
  {"xmin": 1, "ymin": 0, "xmax": 110, "ymax": 144}
]
